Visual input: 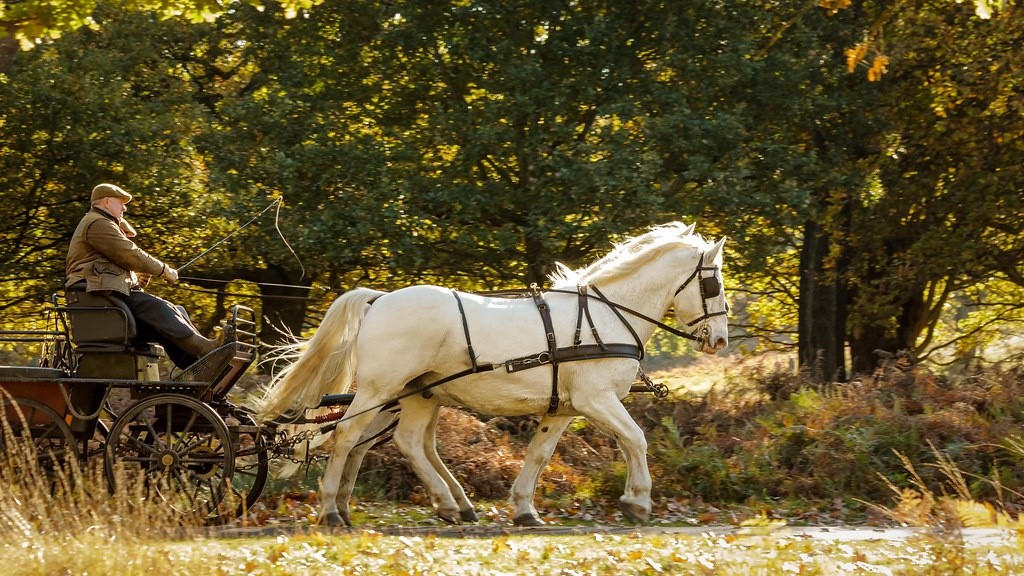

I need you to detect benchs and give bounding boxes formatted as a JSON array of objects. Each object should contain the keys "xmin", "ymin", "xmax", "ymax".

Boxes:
[{"xmin": 44, "ymin": 282, "xmax": 164, "ymax": 357}]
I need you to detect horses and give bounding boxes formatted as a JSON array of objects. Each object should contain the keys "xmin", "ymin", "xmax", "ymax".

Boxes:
[{"xmin": 240, "ymin": 220, "xmax": 729, "ymax": 527}]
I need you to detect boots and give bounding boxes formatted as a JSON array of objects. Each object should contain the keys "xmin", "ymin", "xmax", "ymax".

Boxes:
[{"xmin": 175, "ymin": 325, "xmax": 234, "ymax": 359}]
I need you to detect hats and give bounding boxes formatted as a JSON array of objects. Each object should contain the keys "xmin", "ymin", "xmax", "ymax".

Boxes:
[{"xmin": 90, "ymin": 183, "xmax": 132, "ymax": 204}]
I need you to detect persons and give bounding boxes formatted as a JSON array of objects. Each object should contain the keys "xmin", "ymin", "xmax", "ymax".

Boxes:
[{"xmin": 63, "ymin": 183, "xmax": 234, "ymax": 389}]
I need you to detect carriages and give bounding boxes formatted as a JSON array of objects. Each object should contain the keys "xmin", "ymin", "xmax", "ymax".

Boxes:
[{"xmin": 0, "ymin": 219, "xmax": 731, "ymax": 528}]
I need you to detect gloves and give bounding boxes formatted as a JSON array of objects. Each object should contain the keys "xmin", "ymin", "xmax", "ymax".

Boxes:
[
  {"xmin": 160, "ymin": 263, "xmax": 178, "ymax": 285},
  {"xmin": 135, "ymin": 272, "xmax": 152, "ymax": 288}
]
